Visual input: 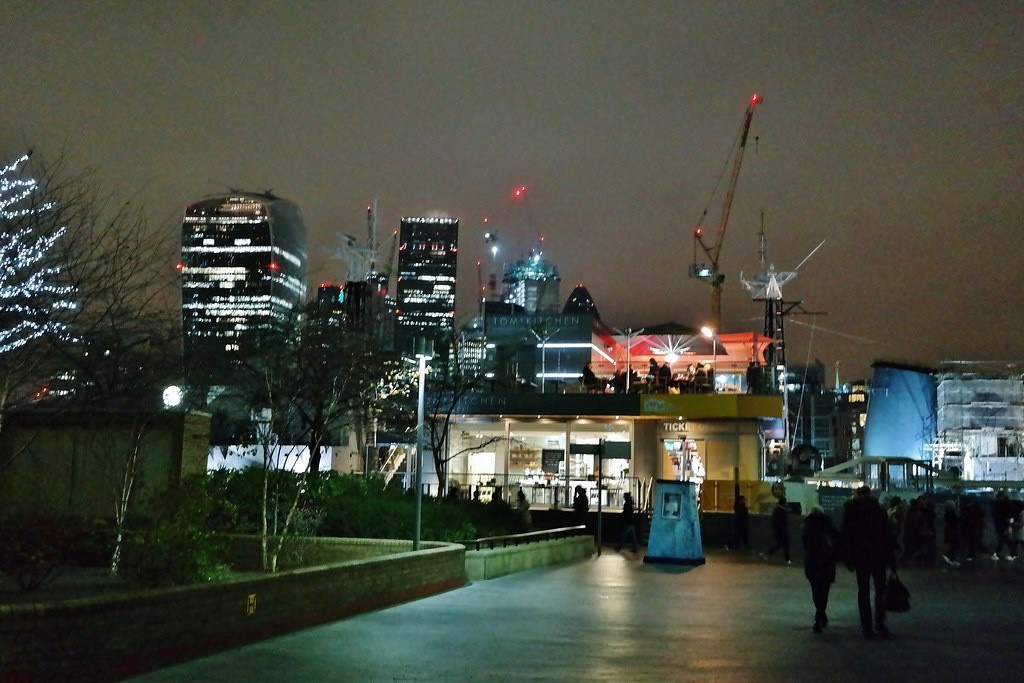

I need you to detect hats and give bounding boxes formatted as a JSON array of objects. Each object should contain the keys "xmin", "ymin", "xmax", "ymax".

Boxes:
[{"xmin": 852, "ymin": 486, "xmax": 870, "ymax": 495}]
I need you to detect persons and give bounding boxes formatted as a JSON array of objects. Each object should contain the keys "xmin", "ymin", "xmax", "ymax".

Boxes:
[
  {"xmin": 583, "ymin": 358, "xmax": 714, "ymax": 394},
  {"xmin": 760, "ymin": 497, "xmax": 792, "ymax": 566},
  {"xmin": 571, "ymin": 486, "xmax": 589, "ymax": 525},
  {"xmin": 838, "ymin": 486, "xmax": 899, "ymax": 641},
  {"xmin": 613, "ymin": 493, "xmax": 638, "ymax": 553},
  {"xmin": 801, "ymin": 506, "xmax": 843, "ymax": 634},
  {"xmin": 746, "ymin": 362, "xmax": 766, "ymax": 394},
  {"xmin": 886, "ymin": 494, "xmax": 1024, "ymax": 570},
  {"xmin": 402, "ymin": 486, "xmax": 533, "ymax": 532},
  {"xmin": 772, "ymin": 483, "xmax": 785, "ymax": 499},
  {"xmin": 725, "ymin": 495, "xmax": 756, "ymax": 554}
]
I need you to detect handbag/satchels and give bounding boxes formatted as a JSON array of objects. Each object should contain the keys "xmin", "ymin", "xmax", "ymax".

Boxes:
[{"xmin": 885, "ymin": 572, "xmax": 911, "ymax": 614}]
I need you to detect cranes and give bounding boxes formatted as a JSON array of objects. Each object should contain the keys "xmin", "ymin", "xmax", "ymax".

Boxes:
[
  {"xmin": 734, "ymin": 208, "xmax": 829, "ymax": 453},
  {"xmin": 691, "ymin": 92, "xmax": 768, "ymax": 336}
]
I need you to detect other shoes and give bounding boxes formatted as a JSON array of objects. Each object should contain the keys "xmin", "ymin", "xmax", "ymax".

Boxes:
[
  {"xmin": 821, "ymin": 618, "xmax": 829, "ymax": 626},
  {"xmin": 813, "ymin": 622, "xmax": 822, "ymax": 633},
  {"xmin": 863, "ymin": 631, "xmax": 874, "ymax": 640},
  {"xmin": 875, "ymin": 624, "xmax": 889, "ymax": 634}
]
